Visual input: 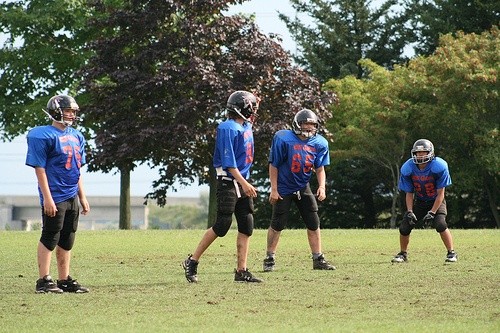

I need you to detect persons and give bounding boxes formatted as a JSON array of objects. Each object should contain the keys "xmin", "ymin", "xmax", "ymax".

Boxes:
[
  {"xmin": 392, "ymin": 139, "xmax": 459, "ymax": 263},
  {"xmin": 262, "ymin": 108, "xmax": 336, "ymax": 272},
  {"xmin": 25, "ymin": 94, "xmax": 91, "ymax": 294},
  {"xmin": 182, "ymin": 90, "xmax": 263, "ymax": 284}
]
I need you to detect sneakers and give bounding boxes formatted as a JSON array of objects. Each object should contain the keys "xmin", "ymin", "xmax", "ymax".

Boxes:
[
  {"xmin": 35, "ymin": 275, "xmax": 63, "ymax": 293},
  {"xmin": 57, "ymin": 275, "xmax": 89, "ymax": 293},
  {"xmin": 445, "ymin": 251, "xmax": 457, "ymax": 262},
  {"xmin": 234, "ymin": 267, "xmax": 262, "ymax": 283},
  {"xmin": 264, "ymin": 253, "xmax": 275, "ymax": 272},
  {"xmin": 313, "ymin": 254, "xmax": 336, "ymax": 270},
  {"xmin": 391, "ymin": 252, "xmax": 407, "ymax": 263},
  {"xmin": 183, "ymin": 253, "xmax": 199, "ymax": 283}
]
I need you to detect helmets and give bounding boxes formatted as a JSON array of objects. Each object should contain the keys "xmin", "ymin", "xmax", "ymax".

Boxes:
[
  {"xmin": 293, "ymin": 109, "xmax": 319, "ymax": 135},
  {"xmin": 412, "ymin": 138, "xmax": 435, "ymax": 164},
  {"xmin": 47, "ymin": 95, "xmax": 80, "ymax": 124},
  {"xmin": 226, "ymin": 91, "xmax": 257, "ymax": 119}
]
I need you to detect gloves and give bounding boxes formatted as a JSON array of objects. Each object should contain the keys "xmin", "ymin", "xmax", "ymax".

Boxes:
[
  {"xmin": 406, "ymin": 212, "xmax": 417, "ymax": 225},
  {"xmin": 424, "ymin": 211, "xmax": 435, "ymax": 224}
]
